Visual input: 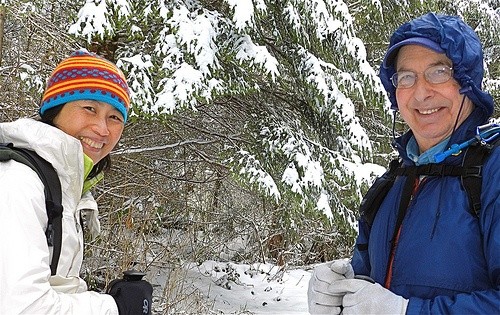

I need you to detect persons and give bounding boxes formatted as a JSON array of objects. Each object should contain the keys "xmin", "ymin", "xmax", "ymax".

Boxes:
[
  {"xmin": 0, "ymin": 47, "xmax": 154, "ymax": 315},
  {"xmin": 306, "ymin": 11, "xmax": 500, "ymax": 315}
]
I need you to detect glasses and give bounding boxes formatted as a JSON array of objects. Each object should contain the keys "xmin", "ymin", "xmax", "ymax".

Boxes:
[{"xmin": 390, "ymin": 65, "xmax": 453, "ymax": 88}]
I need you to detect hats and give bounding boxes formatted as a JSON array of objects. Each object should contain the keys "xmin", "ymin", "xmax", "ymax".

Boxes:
[
  {"xmin": 40, "ymin": 48, "xmax": 129, "ymax": 124},
  {"xmin": 383, "ymin": 37, "xmax": 444, "ymax": 68}
]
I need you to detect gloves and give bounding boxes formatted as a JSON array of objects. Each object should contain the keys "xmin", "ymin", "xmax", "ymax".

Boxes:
[
  {"xmin": 109, "ymin": 280, "xmax": 153, "ymax": 315},
  {"xmin": 307, "ymin": 260, "xmax": 354, "ymax": 315},
  {"xmin": 329, "ymin": 279, "xmax": 409, "ymax": 315}
]
